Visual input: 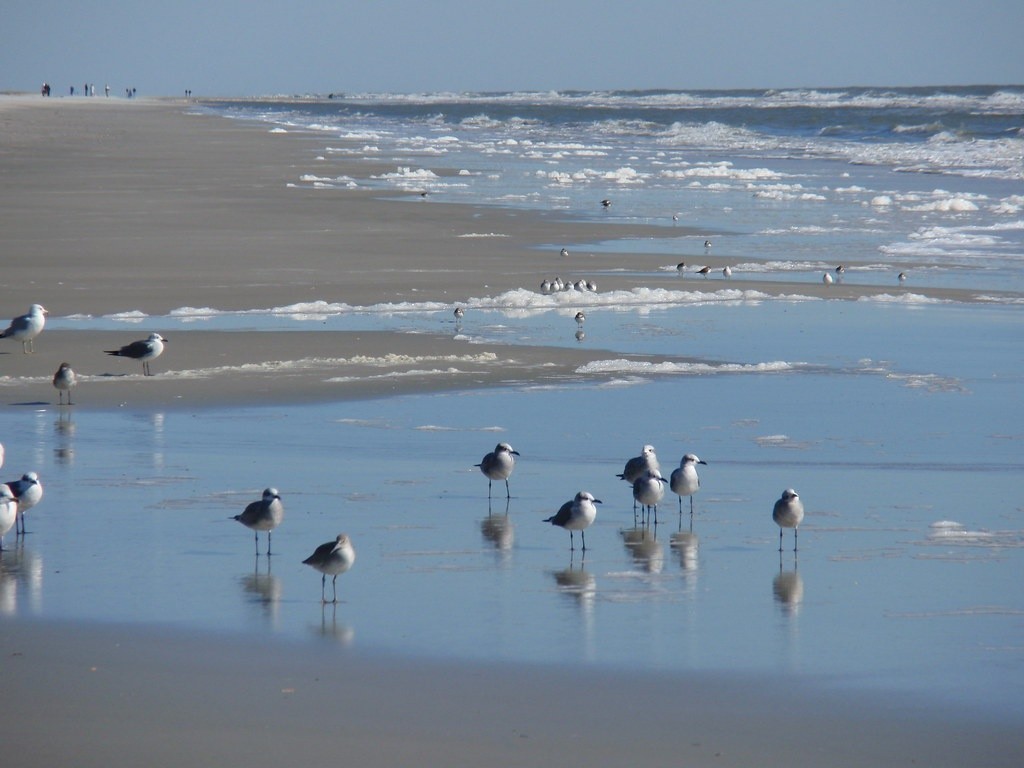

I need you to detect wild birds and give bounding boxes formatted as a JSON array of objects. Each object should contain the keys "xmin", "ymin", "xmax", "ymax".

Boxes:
[
  {"xmin": 616, "ymin": 444, "xmax": 668, "ymax": 523},
  {"xmin": 677, "ymin": 263, "xmax": 732, "ymax": 279},
  {"xmin": 541, "ymin": 277, "xmax": 597, "ymax": 294},
  {"xmin": 303, "ymin": 532, "xmax": 355, "ymax": 603},
  {"xmin": 0, "ymin": 472, "xmax": 44, "ymax": 551},
  {"xmin": 772, "ymin": 488, "xmax": 804, "ymax": 551},
  {"xmin": 227, "ymin": 487, "xmax": 284, "ymax": 554},
  {"xmin": 454, "ymin": 307, "xmax": 464, "ymax": 322},
  {"xmin": 0, "ymin": 303, "xmax": 49, "ymax": 354},
  {"xmin": 670, "ymin": 454, "xmax": 708, "ymax": 514},
  {"xmin": 575, "ymin": 312, "xmax": 586, "ymax": 326},
  {"xmin": 574, "ymin": 327, "xmax": 585, "ymax": 342},
  {"xmin": 673, "ymin": 215, "xmax": 679, "ymax": 221},
  {"xmin": 478, "ymin": 498, "xmax": 806, "ymax": 621},
  {"xmin": 704, "ymin": 240, "xmax": 711, "ymax": 248},
  {"xmin": 53, "ymin": 363, "xmax": 77, "ymax": 405},
  {"xmin": 898, "ymin": 272, "xmax": 907, "ymax": 282},
  {"xmin": 601, "ymin": 199, "xmax": 612, "ymax": 211},
  {"xmin": 560, "ymin": 249, "xmax": 568, "ymax": 258},
  {"xmin": 473, "ymin": 441, "xmax": 521, "ymax": 498},
  {"xmin": 824, "ymin": 266, "xmax": 845, "ymax": 288},
  {"xmin": 543, "ymin": 492, "xmax": 602, "ymax": 549},
  {"xmin": 103, "ymin": 333, "xmax": 168, "ymax": 376},
  {"xmin": 421, "ymin": 192, "xmax": 428, "ymax": 200}
]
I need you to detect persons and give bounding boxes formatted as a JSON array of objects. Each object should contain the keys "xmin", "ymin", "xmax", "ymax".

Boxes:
[
  {"xmin": 185, "ymin": 90, "xmax": 191, "ymax": 96},
  {"xmin": 42, "ymin": 80, "xmax": 136, "ymax": 96}
]
